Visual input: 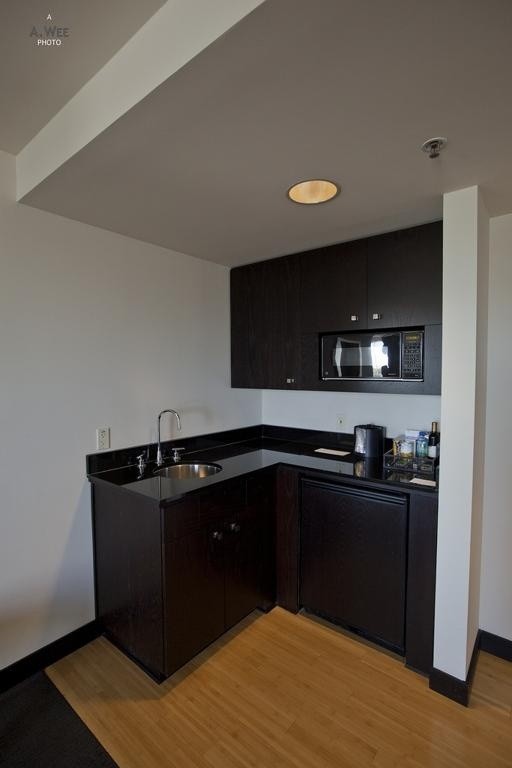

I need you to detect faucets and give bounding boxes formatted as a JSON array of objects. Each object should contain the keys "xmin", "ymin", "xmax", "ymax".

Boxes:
[{"xmin": 154, "ymin": 409, "xmax": 181, "ymax": 467}]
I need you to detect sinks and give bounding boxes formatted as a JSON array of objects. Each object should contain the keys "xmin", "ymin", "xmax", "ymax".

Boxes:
[{"xmin": 152, "ymin": 460, "xmax": 224, "ymax": 482}]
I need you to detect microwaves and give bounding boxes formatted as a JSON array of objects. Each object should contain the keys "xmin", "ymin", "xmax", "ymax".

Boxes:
[{"xmin": 321, "ymin": 324, "xmax": 425, "ymax": 383}]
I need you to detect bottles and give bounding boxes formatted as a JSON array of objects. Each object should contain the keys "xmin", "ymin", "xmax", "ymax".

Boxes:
[
  {"xmin": 416, "ymin": 432, "xmax": 428, "ymax": 458},
  {"xmin": 429, "ymin": 421, "xmax": 440, "ymax": 459}
]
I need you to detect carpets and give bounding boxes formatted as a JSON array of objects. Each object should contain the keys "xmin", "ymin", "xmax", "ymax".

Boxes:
[{"xmin": 1, "ymin": 668, "xmax": 122, "ymax": 767}]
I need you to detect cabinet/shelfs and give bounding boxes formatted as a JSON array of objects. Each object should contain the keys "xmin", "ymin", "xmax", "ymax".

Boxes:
[
  {"xmin": 302, "ymin": 218, "xmax": 442, "ymax": 334},
  {"xmin": 87, "ymin": 463, "xmax": 278, "ymax": 683},
  {"xmin": 230, "ymin": 250, "xmax": 302, "ymax": 391}
]
